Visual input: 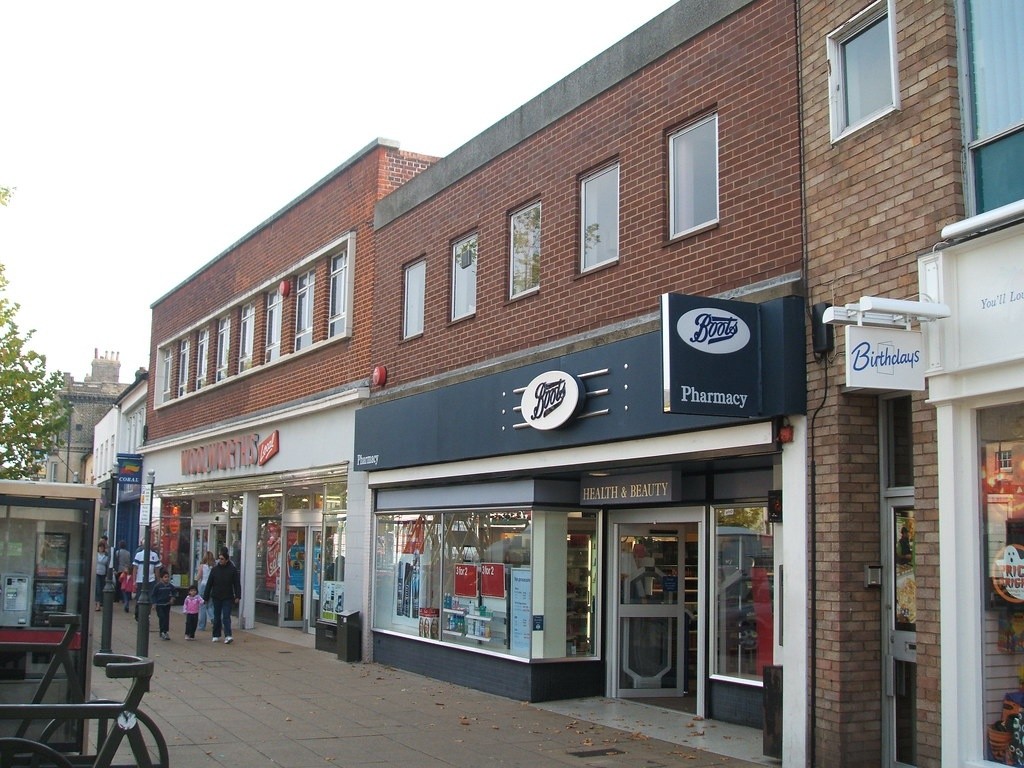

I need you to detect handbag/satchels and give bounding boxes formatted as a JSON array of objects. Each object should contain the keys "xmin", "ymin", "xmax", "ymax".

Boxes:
[{"xmin": 114, "ymin": 550, "xmax": 120, "ymax": 571}]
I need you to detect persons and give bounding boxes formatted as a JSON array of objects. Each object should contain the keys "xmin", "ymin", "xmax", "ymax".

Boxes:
[
  {"xmin": 151, "ymin": 571, "xmax": 179, "ymax": 639},
  {"xmin": 896, "ymin": 526, "xmax": 913, "ymax": 559},
  {"xmin": 132, "ymin": 540, "xmax": 163, "ymax": 622},
  {"xmin": 99, "ymin": 535, "xmax": 109, "ymax": 553},
  {"xmin": 216, "ymin": 547, "xmax": 235, "ymax": 566},
  {"xmin": 194, "ymin": 551, "xmax": 215, "ymax": 630},
  {"xmin": 115, "ymin": 540, "xmax": 131, "ymax": 604},
  {"xmin": 119, "ymin": 567, "xmax": 137, "ymax": 613},
  {"xmin": 182, "ymin": 585, "xmax": 206, "ymax": 640},
  {"xmin": 95, "ymin": 543, "xmax": 108, "ymax": 611},
  {"xmin": 199, "ymin": 552, "xmax": 242, "ymax": 645}
]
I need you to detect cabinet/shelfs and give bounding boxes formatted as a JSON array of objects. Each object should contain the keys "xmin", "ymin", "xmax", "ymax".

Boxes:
[
  {"xmin": 466, "ymin": 615, "xmax": 492, "ymax": 642},
  {"xmin": 442, "ymin": 608, "xmax": 465, "ymax": 636}
]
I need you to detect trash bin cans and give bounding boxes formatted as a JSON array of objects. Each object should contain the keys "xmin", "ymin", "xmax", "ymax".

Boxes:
[{"xmin": 337, "ymin": 610, "xmax": 362, "ymax": 663}]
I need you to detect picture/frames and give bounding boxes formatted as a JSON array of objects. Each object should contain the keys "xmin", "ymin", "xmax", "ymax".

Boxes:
[
  {"xmin": 30, "ymin": 580, "xmax": 68, "ymax": 627},
  {"xmin": 34, "ymin": 532, "xmax": 70, "ymax": 579}
]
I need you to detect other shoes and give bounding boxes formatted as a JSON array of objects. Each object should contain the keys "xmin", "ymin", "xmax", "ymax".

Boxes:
[
  {"xmin": 224, "ymin": 636, "xmax": 233, "ymax": 644},
  {"xmin": 133, "ymin": 614, "xmax": 138, "ymax": 623},
  {"xmin": 185, "ymin": 634, "xmax": 189, "ymax": 640},
  {"xmin": 160, "ymin": 633, "xmax": 167, "ymax": 639},
  {"xmin": 165, "ymin": 632, "xmax": 170, "ymax": 640},
  {"xmin": 212, "ymin": 637, "xmax": 219, "ymax": 642}
]
